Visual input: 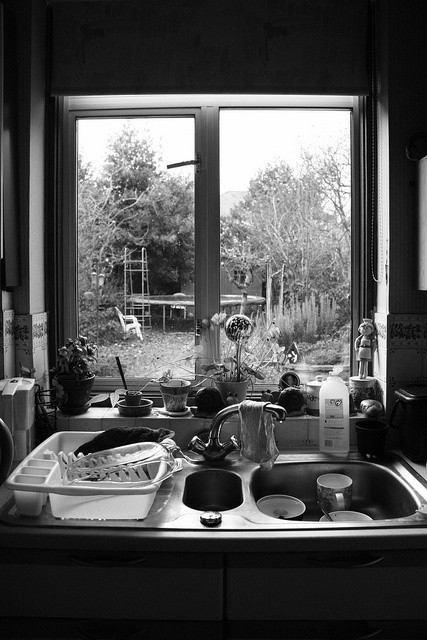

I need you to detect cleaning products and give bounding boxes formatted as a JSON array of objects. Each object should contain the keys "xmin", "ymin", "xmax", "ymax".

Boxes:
[{"xmin": 319, "ymin": 373, "xmax": 349, "ymax": 456}]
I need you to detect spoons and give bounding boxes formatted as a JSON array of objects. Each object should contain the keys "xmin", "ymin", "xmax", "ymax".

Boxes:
[{"xmin": 317, "ymin": 500, "xmax": 332, "ymax": 521}]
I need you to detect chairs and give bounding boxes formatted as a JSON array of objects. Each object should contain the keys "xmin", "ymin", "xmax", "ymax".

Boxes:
[
  {"xmin": 115, "ymin": 307, "xmax": 144, "ymax": 341},
  {"xmin": 169, "ymin": 293, "xmax": 187, "ymax": 321}
]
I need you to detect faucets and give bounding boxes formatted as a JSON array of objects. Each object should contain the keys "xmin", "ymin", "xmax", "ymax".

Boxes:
[{"xmin": 188, "ymin": 401, "xmax": 287, "ymax": 461}]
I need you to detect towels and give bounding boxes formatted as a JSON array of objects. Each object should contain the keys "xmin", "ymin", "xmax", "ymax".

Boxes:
[{"xmin": 238, "ymin": 400, "xmax": 280, "ymax": 472}]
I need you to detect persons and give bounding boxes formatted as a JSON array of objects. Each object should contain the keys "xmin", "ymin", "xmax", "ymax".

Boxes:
[{"xmin": 354, "ymin": 318, "xmax": 376, "ymax": 379}]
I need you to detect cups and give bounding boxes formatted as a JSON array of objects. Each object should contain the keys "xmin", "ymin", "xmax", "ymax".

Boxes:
[
  {"xmin": 317, "ymin": 473, "xmax": 354, "ymax": 510},
  {"xmin": 355, "ymin": 419, "xmax": 385, "ymax": 456}
]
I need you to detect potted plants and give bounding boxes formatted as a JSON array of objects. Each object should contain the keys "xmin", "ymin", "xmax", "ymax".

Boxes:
[{"xmin": 52, "ymin": 334, "xmax": 95, "ymax": 415}]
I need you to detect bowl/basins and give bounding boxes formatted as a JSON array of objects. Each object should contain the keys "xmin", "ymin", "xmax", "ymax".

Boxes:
[
  {"xmin": 0, "ymin": 419, "xmax": 14, "ymax": 487},
  {"xmin": 256, "ymin": 494, "xmax": 306, "ymax": 519},
  {"xmin": 320, "ymin": 510, "xmax": 374, "ymax": 521}
]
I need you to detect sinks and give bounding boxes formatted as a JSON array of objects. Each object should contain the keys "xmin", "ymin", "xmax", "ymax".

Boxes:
[
  {"xmin": 250, "ymin": 460, "xmax": 416, "ymax": 528},
  {"xmin": 180, "ymin": 465, "xmax": 245, "ymax": 513}
]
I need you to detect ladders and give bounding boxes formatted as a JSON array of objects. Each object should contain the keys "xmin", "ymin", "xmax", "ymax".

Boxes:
[{"xmin": 123, "ymin": 248, "xmax": 151, "ymax": 335}]
